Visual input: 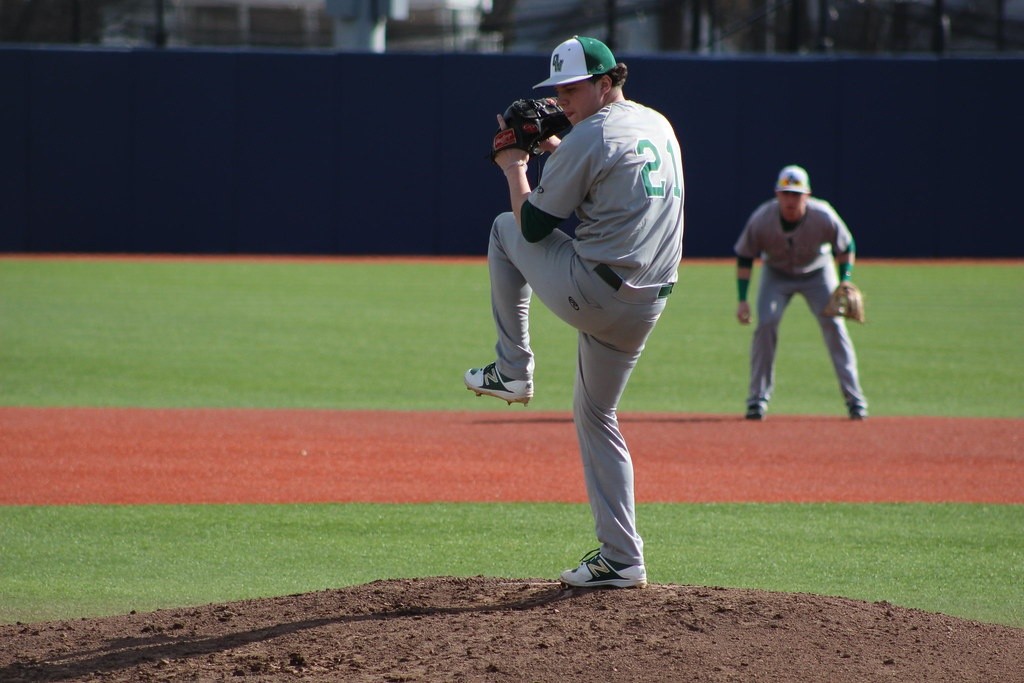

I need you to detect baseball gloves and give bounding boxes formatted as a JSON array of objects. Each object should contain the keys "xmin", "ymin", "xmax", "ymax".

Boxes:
[
  {"xmin": 490, "ymin": 96, "xmax": 569, "ymax": 157},
  {"xmin": 821, "ymin": 283, "xmax": 864, "ymax": 321}
]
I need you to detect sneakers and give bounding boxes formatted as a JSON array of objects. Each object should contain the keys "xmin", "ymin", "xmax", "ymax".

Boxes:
[
  {"xmin": 558, "ymin": 554, "xmax": 647, "ymax": 589},
  {"xmin": 463, "ymin": 362, "xmax": 534, "ymax": 408}
]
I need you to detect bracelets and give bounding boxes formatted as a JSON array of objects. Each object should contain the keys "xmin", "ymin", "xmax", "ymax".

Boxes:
[{"xmin": 504, "ymin": 161, "xmax": 528, "ymax": 176}]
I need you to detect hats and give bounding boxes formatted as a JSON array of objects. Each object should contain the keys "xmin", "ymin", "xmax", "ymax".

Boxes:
[
  {"xmin": 775, "ymin": 164, "xmax": 811, "ymax": 196},
  {"xmin": 531, "ymin": 35, "xmax": 618, "ymax": 90}
]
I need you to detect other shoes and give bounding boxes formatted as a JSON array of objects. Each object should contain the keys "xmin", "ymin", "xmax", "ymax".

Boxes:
[
  {"xmin": 851, "ymin": 407, "xmax": 865, "ymax": 420},
  {"xmin": 745, "ymin": 405, "xmax": 764, "ymax": 419}
]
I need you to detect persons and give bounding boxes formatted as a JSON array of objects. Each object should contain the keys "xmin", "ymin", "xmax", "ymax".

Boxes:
[
  {"xmin": 733, "ymin": 164, "xmax": 869, "ymax": 421},
  {"xmin": 464, "ymin": 35, "xmax": 685, "ymax": 590}
]
